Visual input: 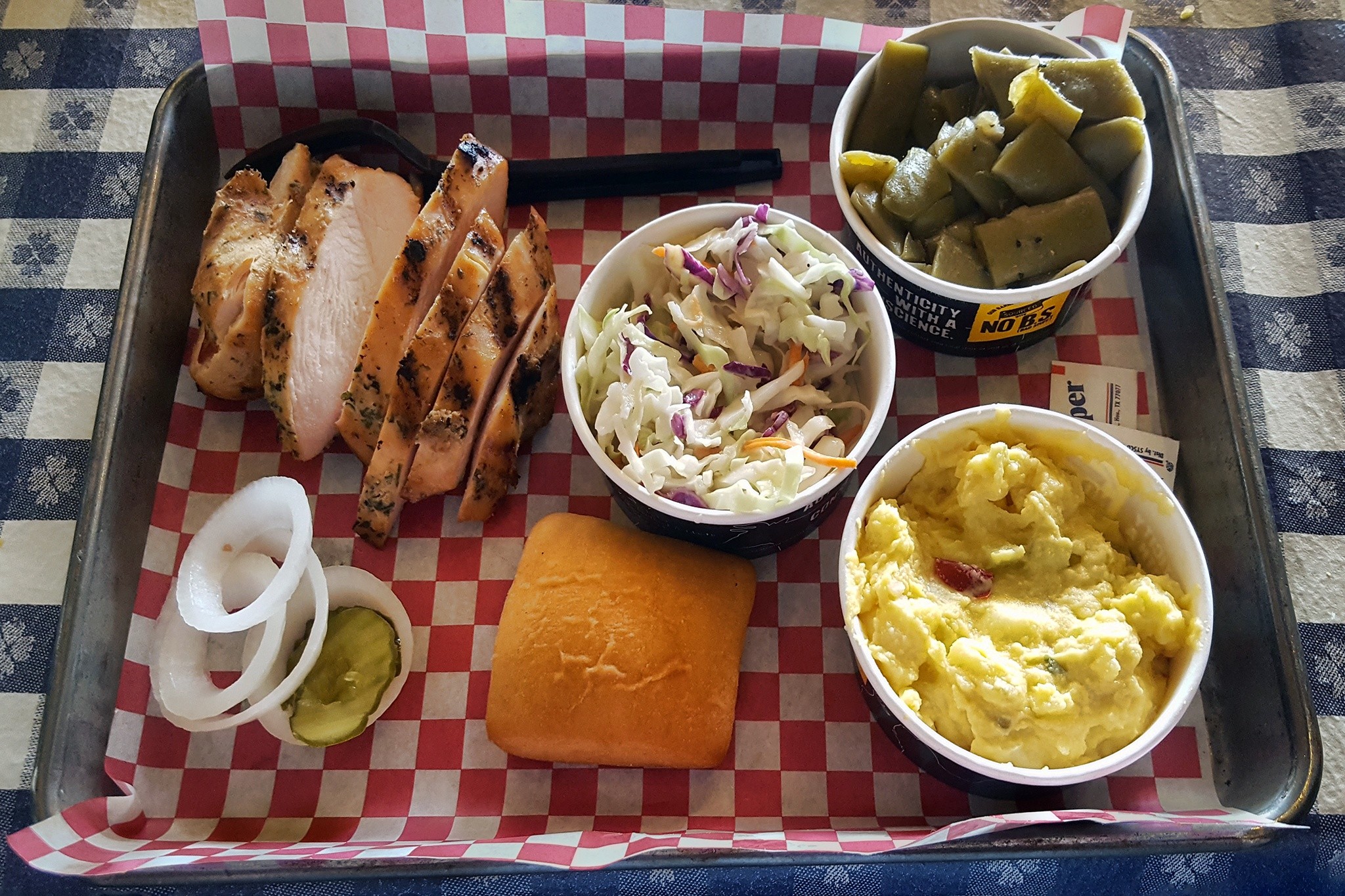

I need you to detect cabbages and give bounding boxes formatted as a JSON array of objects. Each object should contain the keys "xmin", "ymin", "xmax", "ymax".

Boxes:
[{"xmin": 576, "ymin": 204, "xmax": 877, "ymax": 511}]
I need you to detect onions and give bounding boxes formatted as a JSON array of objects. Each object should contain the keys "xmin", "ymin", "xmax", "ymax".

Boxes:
[{"xmin": 147, "ymin": 476, "xmax": 414, "ymax": 746}]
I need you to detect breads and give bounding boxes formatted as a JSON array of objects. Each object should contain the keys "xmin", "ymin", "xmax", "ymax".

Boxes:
[{"xmin": 484, "ymin": 512, "xmax": 755, "ymax": 765}]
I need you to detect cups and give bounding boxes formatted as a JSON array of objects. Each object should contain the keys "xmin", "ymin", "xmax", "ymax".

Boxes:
[
  {"xmin": 563, "ymin": 200, "xmax": 903, "ymax": 559},
  {"xmin": 841, "ymin": 402, "xmax": 1217, "ymax": 796},
  {"xmin": 827, "ymin": 13, "xmax": 1155, "ymax": 360}
]
